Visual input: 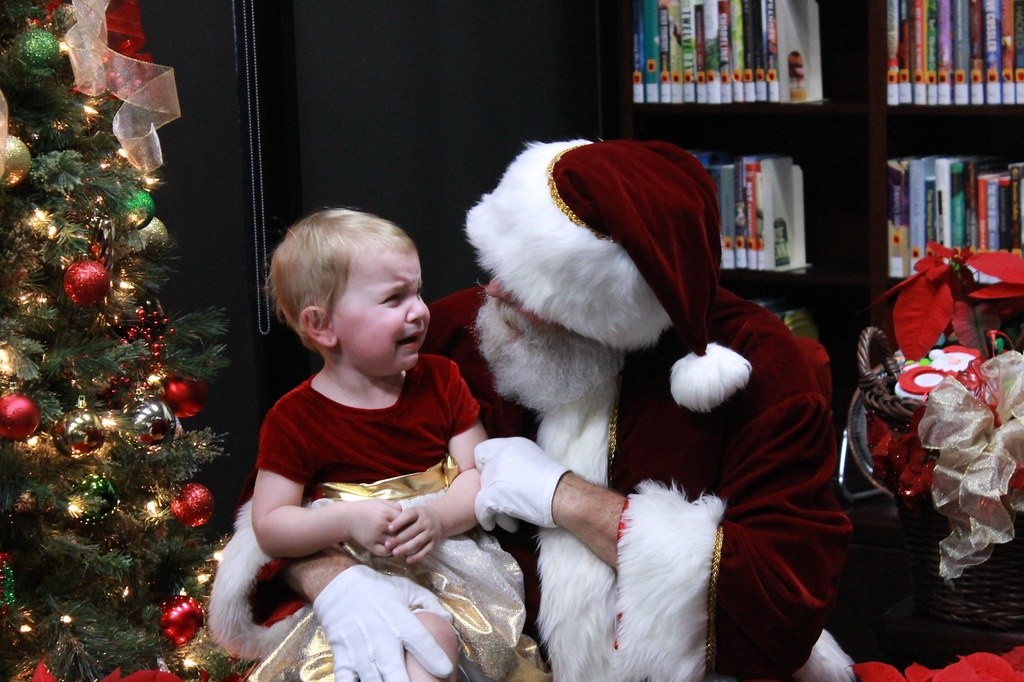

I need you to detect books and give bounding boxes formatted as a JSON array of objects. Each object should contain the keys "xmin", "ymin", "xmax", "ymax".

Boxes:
[
  {"xmin": 888, "ymin": 156, "xmax": 1022, "ymax": 282},
  {"xmin": 686, "ymin": 148, "xmax": 815, "ymax": 276},
  {"xmin": 630, "ymin": 1, "xmax": 822, "ymax": 103},
  {"xmin": 885, "ymin": 0, "xmax": 1024, "ymax": 105}
]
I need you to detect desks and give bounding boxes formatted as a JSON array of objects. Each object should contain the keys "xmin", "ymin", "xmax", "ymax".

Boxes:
[{"xmin": 842, "ymin": 514, "xmax": 1024, "ymax": 682}]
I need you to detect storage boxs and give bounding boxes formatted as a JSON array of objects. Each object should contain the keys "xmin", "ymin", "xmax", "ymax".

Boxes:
[{"xmin": 858, "ymin": 323, "xmax": 1023, "ymax": 629}]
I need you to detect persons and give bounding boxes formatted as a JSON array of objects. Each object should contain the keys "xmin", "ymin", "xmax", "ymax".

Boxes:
[{"xmin": 204, "ymin": 133, "xmax": 862, "ymax": 682}]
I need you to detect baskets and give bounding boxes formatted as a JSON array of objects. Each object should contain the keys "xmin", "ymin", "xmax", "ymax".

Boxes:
[{"xmin": 858, "ymin": 328, "xmax": 1023, "ymax": 630}]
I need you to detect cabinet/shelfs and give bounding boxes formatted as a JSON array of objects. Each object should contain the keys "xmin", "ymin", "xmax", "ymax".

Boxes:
[{"xmin": 592, "ymin": 1, "xmax": 1023, "ymax": 484}]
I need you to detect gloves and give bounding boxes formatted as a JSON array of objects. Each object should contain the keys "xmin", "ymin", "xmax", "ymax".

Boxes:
[
  {"xmin": 471, "ymin": 436, "xmax": 573, "ymax": 534},
  {"xmin": 312, "ymin": 564, "xmax": 454, "ymax": 682}
]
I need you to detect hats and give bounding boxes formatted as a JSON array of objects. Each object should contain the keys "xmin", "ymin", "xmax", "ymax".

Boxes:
[{"xmin": 461, "ymin": 140, "xmax": 749, "ymax": 413}]
